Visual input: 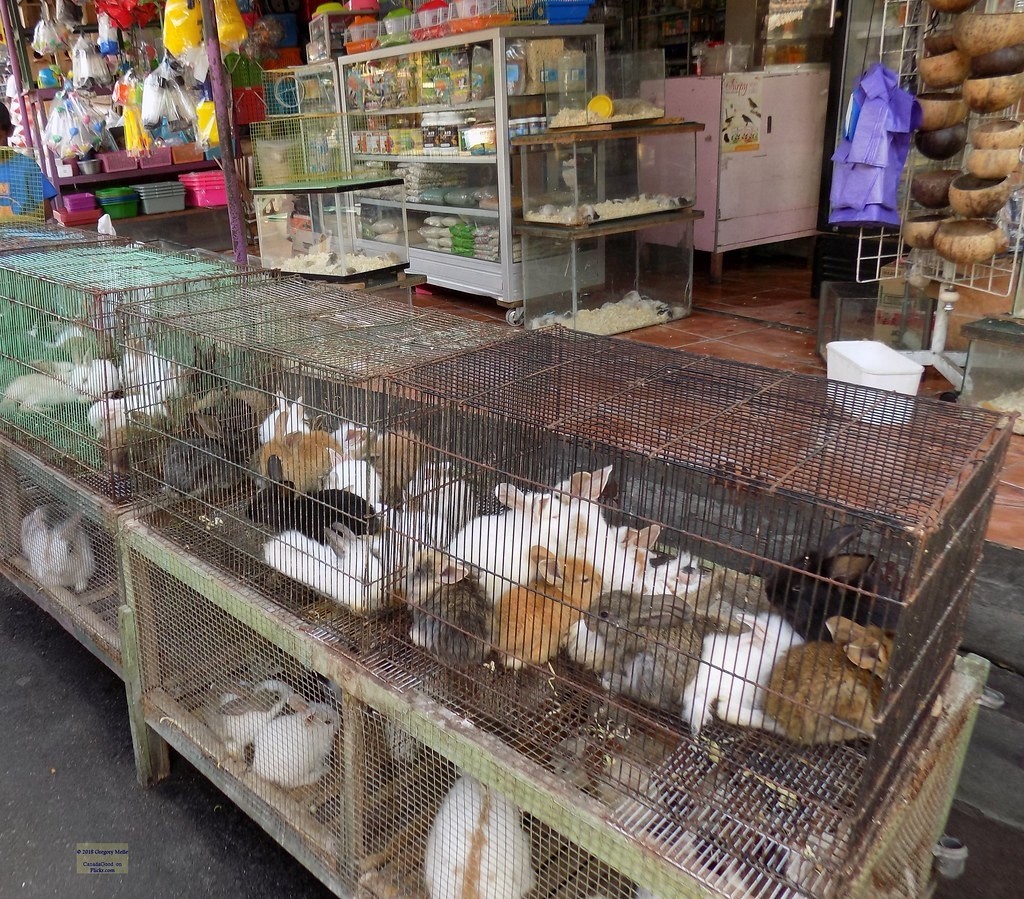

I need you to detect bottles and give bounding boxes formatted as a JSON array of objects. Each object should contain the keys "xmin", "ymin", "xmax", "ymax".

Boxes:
[{"xmin": 388, "ymin": 111, "xmax": 548, "ymax": 156}]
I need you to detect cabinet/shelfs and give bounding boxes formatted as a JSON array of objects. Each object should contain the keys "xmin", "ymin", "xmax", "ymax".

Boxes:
[
  {"xmin": 339, "ymin": 23, "xmax": 606, "ymax": 308},
  {"xmin": 1, "ymin": 0, "xmax": 238, "ymax": 226},
  {"xmin": 632, "ymin": 0, "xmax": 727, "ymax": 77}
]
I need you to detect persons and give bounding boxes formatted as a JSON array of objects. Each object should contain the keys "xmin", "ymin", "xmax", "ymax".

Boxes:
[{"xmin": 0, "ymin": 102, "xmax": 59, "ymax": 225}]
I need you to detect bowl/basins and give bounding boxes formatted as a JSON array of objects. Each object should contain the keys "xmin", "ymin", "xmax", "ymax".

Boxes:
[
  {"xmin": 76, "ymin": 159, "xmax": 100, "ymax": 174},
  {"xmin": 900, "ymin": 0, "xmax": 1024, "ymax": 265}
]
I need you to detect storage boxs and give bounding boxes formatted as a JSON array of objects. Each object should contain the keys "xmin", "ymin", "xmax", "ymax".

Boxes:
[
  {"xmin": 814, "ymin": 281, "xmax": 932, "ymax": 363},
  {"xmin": 510, "ymin": 47, "xmax": 706, "ymax": 333},
  {"xmin": 959, "ymin": 319, "xmax": 1024, "ymax": 436},
  {"xmin": 825, "ymin": 340, "xmax": 925, "ymax": 425},
  {"xmin": 249, "ymin": 177, "xmax": 410, "ymax": 280},
  {"xmin": 876, "ymin": 258, "xmax": 1021, "ymax": 351}
]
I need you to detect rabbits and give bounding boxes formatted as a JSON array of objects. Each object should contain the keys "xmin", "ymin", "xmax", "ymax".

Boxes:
[
  {"xmin": 5, "ymin": 310, "xmax": 300, "ymax": 454},
  {"xmin": 204, "ymin": 669, "xmax": 424, "ymax": 791},
  {"xmin": 427, "ymin": 765, "xmax": 537, "ymax": 899},
  {"xmin": 20, "ymin": 503, "xmax": 103, "ymax": 595},
  {"xmin": 240, "ymin": 391, "xmax": 475, "ymax": 619},
  {"xmin": 434, "ymin": 458, "xmax": 918, "ymax": 744}
]
